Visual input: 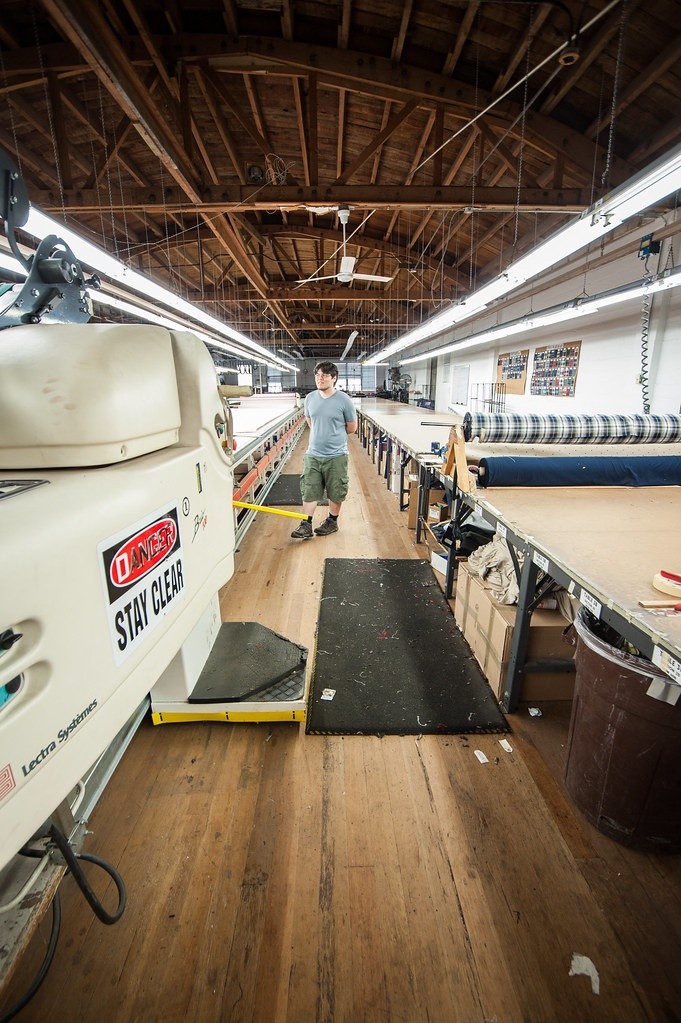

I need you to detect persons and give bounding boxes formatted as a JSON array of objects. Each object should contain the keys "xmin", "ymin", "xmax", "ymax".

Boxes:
[{"xmin": 290, "ymin": 360, "xmax": 357, "ymax": 538}]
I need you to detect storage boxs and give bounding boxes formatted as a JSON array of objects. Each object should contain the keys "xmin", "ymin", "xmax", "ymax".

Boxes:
[
  {"xmin": 408, "ymin": 481, "xmax": 448, "ymax": 529},
  {"xmin": 454, "ymin": 563, "xmax": 577, "ymax": 712},
  {"xmin": 428, "ymin": 502, "xmax": 448, "ymax": 525}
]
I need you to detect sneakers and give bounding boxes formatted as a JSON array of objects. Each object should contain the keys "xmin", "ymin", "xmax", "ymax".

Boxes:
[
  {"xmin": 290, "ymin": 520, "xmax": 313, "ymax": 538},
  {"xmin": 313, "ymin": 518, "xmax": 338, "ymax": 535}
]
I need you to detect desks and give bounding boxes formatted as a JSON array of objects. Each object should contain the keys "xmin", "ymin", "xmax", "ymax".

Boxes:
[{"xmin": 355, "ymin": 395, "xmax": 681, "ymax": 708}]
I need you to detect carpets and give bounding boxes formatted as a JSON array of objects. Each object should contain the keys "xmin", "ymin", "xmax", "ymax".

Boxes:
[
  {"xmin": 262, "ymin": 472, "xmax": 329, "ymax": 506},
  {"xmin": 304, "ymin": 559, "xmax": 513, "ymax": 736}
]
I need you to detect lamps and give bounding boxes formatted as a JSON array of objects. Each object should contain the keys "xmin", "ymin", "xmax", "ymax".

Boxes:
[
  {"xmin": 360, "ymin": 0, "xmax": 681, "ymax": 369},
  {"xmin": 0, "ymin": 0, "xmax": 302, "ymax": 373}
]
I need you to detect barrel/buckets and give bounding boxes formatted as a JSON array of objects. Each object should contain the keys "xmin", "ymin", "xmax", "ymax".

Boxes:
[{"xmin": 562, "ymin": 604, "xmax": 681, "ymax": 856}]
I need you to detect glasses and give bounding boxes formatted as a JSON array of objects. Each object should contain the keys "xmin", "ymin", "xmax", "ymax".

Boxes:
[{"xmin": 315, "ymin": 374, "xmax": 331, "ymax": 378}]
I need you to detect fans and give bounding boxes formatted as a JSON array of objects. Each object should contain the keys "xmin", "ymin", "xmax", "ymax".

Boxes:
[
  {"xmin": 399, "ymin": 374, "xmax": 412, "ymax": 399},
  {"xmin": 294, "ymin": 206, "xmax": 393, "ymax": 284}
]
